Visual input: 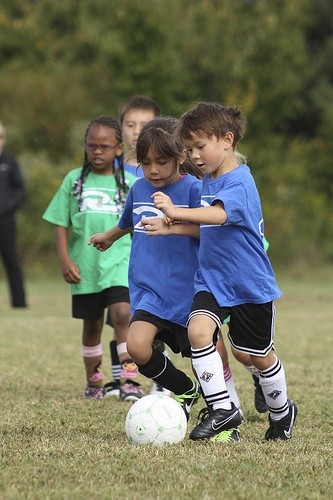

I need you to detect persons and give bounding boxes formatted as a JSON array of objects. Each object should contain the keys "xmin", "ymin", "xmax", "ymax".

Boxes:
[
  {"xmin": 0, "ymin": 124, "xmax": 28, "ymax": 308},
  {"xmin": 41, "ymin": 96, "xmax": 296, "ymax": 444}
]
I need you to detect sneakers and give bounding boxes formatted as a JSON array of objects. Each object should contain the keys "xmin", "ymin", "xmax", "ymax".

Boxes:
[
  {"xmin": 265, "ymin": 399, "xmax": 298, "ymax": 441},
  {"xmin": 172, "ymin": 374, "xmax": 201, "ymax": 422},
  {"xmin": 209, "ymin": 427, "xmax": 240, "ymax": 442},
  {"xmin": 251, "ymin": 374, "xmax": 268, "ymax": 413},
  {"xmin": 189, "ymin": 402, "xmax": 241, "ymax": 439}
]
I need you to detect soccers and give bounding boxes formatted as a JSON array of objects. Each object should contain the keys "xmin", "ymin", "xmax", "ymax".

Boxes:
[{"xmin": 125, "ymin": 393, "xmax": 188, "ymax": 446}]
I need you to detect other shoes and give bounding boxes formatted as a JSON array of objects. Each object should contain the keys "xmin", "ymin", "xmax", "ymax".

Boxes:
[
  {"xmin": 84, "ymin": 386, "xmax": 103, "ymax": 399},
  {"xmin": 149, "ymin": 382, "xmax": 170, "ymax": 396},
  {"xmin": 106, "ymin": 380, "xmax": 121, "ymax": 397},
  {"xmin": 119, "ymin": 379, "xmax": 141, "ymax": 401}
]
